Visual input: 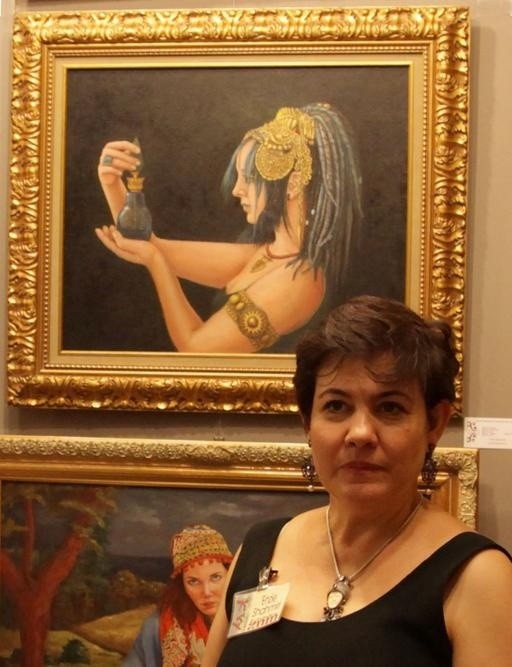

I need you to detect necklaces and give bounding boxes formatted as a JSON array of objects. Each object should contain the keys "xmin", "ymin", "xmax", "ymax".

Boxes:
[
  {"xmin": 250, "ymin": 237, "xmax": 305, "ymax": 272},
  {"xmin": 320, "ymin": 490, "xmax": 423, "ymax": 622}
]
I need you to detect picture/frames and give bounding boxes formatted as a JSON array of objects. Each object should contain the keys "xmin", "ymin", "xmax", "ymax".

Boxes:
[
  {"xmin": 0, "ymin": 434, "xmax": 479, "ymax": 667},
  {"xmin": 6, "ymin": 4, "xmax": 472, "ymax": 421}
]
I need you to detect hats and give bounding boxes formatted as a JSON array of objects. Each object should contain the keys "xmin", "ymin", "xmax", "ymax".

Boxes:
[{"xmin": 167, "ymin": 523, "xmax": 232, "ymax": 579}]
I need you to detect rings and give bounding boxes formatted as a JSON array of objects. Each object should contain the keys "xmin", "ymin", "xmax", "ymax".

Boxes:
[{"xmin": 101, "ymin": 154, "xmax": 113, "ymax": 168}]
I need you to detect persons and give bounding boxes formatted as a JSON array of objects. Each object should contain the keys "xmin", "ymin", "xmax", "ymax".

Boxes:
[
  {"xmin": 196, "ymin": 293, "xmax": 512, "ymax": 667},
  {"xmin": 94, "ymin": 100, "xmax": 365, "ymax": 358},
  {"xmin": 119, "ymin": 525, "xmax": 235, "ymax": 666}
]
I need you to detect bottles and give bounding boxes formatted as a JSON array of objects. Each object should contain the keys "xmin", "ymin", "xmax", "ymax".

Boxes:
[{"xmin": 115, "ymin": 176, "xmax": 153, "ymax": 243}]
